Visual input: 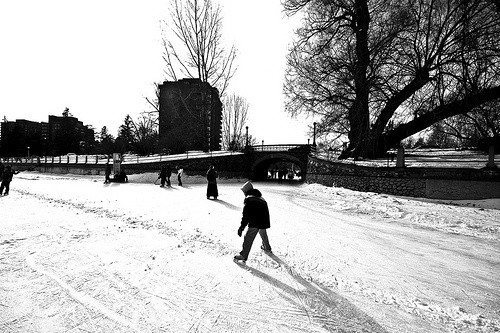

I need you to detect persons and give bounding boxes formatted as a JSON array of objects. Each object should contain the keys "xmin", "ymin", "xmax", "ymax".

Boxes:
[
  {"xmin": 268, "ymin": 163, "xmax": 300, "ymax": 183},
  {"xmin": 104, "ymin": 162, "xmax": 110, "ymax": 184},
  {"xmin": 0, "ymin": 161, "xmax": 15, "ymax": 196},
  {"xmin": 110, "ymin": 169, "xmax": 128, "ymax": 183},
  {"xmin": 175, "ymin": 165, "xmax": 185, "ymax": 186},
  {"xmin": 165, "ymin": 164, "xmax": 173, "ymax": 186},
  {"xmin": 234, "ymin": 180, "xmax": 273, "ymax": 261},
  {"xmin": 205, "ymin": 164, "xmax": 219, "ymax": 200},
  {"xmin": 159, "ymin": 164, "xmax": 167, "ymax": 187}
]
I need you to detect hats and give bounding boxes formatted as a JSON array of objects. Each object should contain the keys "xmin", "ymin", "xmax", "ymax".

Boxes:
[{"xmin": 241, "ymin": 181, "xmax": 253, "ymax": 191}]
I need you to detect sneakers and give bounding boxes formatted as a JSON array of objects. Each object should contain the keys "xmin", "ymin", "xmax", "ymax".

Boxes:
[
  {"xmin": 261, "ymin": 245, "xmax": 272, "ymax": 253},
  {"xmin": 233, "ymin": 255, "xmax": 248, "ymax": 266}
]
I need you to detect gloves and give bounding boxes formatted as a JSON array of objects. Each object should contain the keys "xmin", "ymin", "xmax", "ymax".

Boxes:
[{"xmin": 238, "ymin": 228, "xmax": 243, "ymax": 236}]
